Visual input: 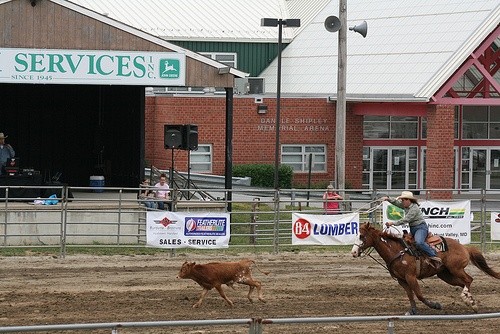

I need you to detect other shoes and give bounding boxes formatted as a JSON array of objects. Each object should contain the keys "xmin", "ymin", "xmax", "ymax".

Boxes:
[{"xmin": 436, "ymin": 260, "xmax": 443, "ymax": 269}]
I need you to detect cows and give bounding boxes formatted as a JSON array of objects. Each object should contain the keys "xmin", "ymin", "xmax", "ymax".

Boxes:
[{"xmin": 175, "ymin": 259, "xmax": 271, "ymax": 309}]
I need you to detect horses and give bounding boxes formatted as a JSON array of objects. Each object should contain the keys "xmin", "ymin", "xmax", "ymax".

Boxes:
[{"xmin": 351, "ymin": 221, "xmax": 500, "ymax": 317}]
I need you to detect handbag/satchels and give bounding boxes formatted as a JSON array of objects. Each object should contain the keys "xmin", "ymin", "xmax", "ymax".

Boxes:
[
  {"xmin": 138, "ymin": 193, "xmax": 148, "ymax": 202},
  {"xmin": 46, "ymin": 194, "xmax": 58, "ymax": 206},
  {"xmin": 34, "ymin": 197, "xmax": 45, "ymax": 205}
]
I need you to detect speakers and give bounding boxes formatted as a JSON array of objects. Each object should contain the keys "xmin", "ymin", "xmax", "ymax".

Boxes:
[{"xmin": 163, "ymin": 124, "xmax": 199, "ymax": 151}]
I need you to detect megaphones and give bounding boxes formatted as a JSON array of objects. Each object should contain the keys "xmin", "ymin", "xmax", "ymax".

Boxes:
[
  {"xmin": 349, "ymin": 19, "xmax": 368, "ymax": 39},
  {"xmin": 323, "ymin": 15, "xmax": 341, "ymax": 32}
]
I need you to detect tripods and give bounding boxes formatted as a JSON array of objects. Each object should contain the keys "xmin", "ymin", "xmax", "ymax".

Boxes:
[{"xmin": 167, "ymin": 148, "xmax": 207, "ymax": 201}]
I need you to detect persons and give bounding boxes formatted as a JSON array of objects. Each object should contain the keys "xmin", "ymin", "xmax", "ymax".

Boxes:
[
  {"xmin": 0, "ymin": 133, "xmax": 16, "ymax": 165},
  {"xmin": 383, "ymin": 190, "xmax": 441, "ymax": 269},
  {"xmin": 324, "ymin": 185, "xmax": 344, "ymax": 215},
  {"xmin": 153, "ymin": 173, "xmax": 171, "ymax": 210},
  {"xmin": 137, "ymin": 179, "xmax": 155, "ymax": 211}
]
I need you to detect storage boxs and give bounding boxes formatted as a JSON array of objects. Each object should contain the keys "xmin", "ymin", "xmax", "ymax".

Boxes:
[{"xmin": 90, "ymin": 174, "xmax": 105, "ymax": 193}]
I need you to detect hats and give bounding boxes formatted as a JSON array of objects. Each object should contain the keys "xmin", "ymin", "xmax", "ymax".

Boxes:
[
  {"xmin": 398, "ymin": 191, "xmax": 418, "ymax": 200},
  {"xmin": 327, "ymin": 185, "xmax": 333, "ymax": 188},
  {"xmin": 0, "ymin": 133, "xmax": 9, "ymax": 138}
]
